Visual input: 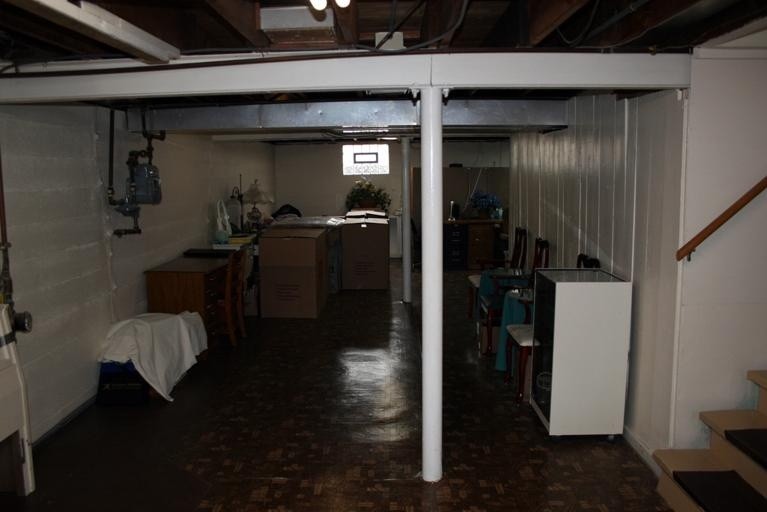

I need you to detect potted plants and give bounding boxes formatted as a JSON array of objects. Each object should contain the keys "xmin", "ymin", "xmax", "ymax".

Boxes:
[{"xmin": 345, "ymin": 176, "xmax": 393, "ymax": 214}]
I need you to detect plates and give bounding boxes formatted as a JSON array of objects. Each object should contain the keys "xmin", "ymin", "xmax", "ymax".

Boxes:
[{"xmin": 509, "ymin": 289, "xmax": 532, "ymax": 301}]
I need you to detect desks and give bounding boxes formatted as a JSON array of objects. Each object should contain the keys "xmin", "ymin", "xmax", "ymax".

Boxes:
[{"xmin": 144, "ymin": 251, "xmax": 243, "ymax": 362}]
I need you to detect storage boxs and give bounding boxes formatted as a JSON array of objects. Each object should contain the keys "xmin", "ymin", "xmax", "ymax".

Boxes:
[{"xmin": 244, "ymin": 207, "xmax": 391, "ymax": 321}]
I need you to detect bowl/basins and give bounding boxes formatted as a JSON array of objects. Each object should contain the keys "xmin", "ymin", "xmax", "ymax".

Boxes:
[
  {"xmin": 214, "ymin": 230, "xmax": 231, "ymax": 244},
  {"xmin": 228, "ymin": 234, "xmax": 256, "ymax": 244}
]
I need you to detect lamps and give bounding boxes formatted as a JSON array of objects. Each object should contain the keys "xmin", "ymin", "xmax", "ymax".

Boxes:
[{"xmin": 241, "ymin": 177, "xmax": 274, "ymax": 221}]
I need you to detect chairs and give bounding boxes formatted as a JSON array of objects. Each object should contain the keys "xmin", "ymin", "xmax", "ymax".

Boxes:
[
  {"xmin": 216, "ymin": 247, "xmax": 246, "ymax": 350},
  {"xmin": 468, "ymin": 222, "xmax": 601, "ymax": 404}
]
